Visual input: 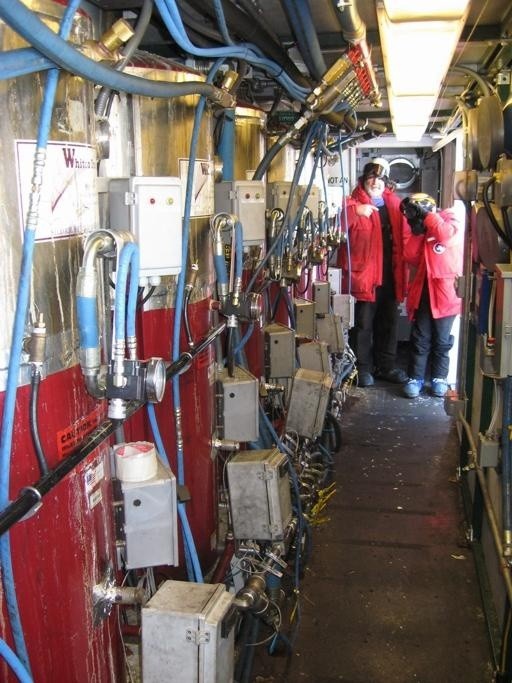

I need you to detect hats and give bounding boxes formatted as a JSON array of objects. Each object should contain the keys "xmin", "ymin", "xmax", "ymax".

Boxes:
[{"xmin": 363, "ymin": 163, "xmax": 389, "ymax": 185}]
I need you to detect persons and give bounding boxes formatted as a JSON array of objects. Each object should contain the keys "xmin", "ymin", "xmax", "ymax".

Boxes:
[
  {"xmin": 336, "ymin": 156, "xmax": 412, "ymax": 387},
  {"xmin": 398, "ymin": 192, "xmax": 465, "ymax": 399}
]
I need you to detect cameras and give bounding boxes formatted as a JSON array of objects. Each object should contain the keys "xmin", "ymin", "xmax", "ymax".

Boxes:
[{"xmin": 399, "ymin": 196, "xmax": 419, "ymax": 221}]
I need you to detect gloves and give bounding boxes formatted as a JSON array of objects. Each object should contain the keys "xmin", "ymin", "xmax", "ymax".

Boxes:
[
  {"xmin": 405, "ymin": 204, "xmax": 427, "ymax": 219},
  {"xmin": 407, "ymin": 218, "xmax": 427, "ymax": 235}
]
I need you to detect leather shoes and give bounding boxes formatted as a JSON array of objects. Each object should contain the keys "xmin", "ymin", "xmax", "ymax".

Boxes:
[
  {"xmin": 401, "ymin": 380, "xmax": 422, "ymax": 398},
  {"xmin": 430, "ymin": 378, "xmax": 448, "ymax": 396},
  {"xmin": 383, "ymin": 368, "xmax": 409, "ymax": 382},
  {"xmin": 356, "ymin": 372, "xmax": 374, "ymax": 386}
]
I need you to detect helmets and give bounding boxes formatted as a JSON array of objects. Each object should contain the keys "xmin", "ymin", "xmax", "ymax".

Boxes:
[{"xmin": 399, "ymin": 193, "xmax": 437, "ymax": 218}]
